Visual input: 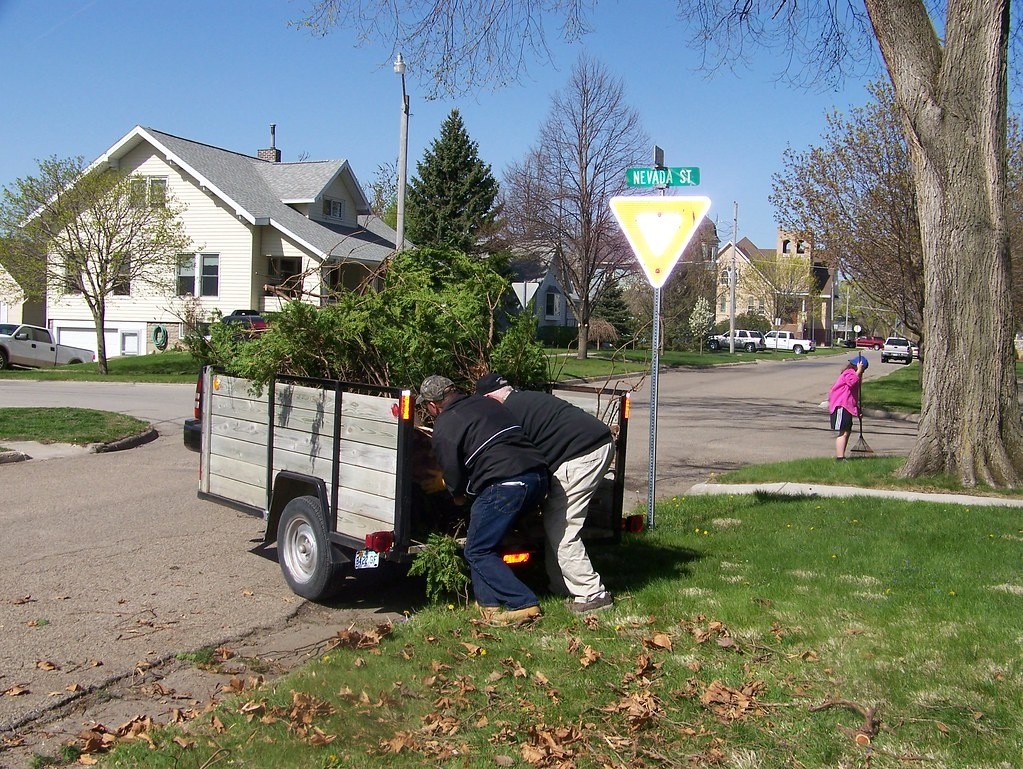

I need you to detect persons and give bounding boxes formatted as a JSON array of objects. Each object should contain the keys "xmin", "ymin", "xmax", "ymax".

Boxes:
[
  {"xmin": 416, "ymin": 374, "xmax": 541, "ymax": 620},
  {"xmin": 829, "ymin": 356, "xmax": 869, "ymax": 460},
  {"xmin": 475, "ymin": 373, "xmax": 614, "ymax": 614}
]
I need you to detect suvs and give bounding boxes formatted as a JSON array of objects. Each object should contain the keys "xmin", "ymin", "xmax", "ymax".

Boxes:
[
  {"xmin": 707, "ymin": 329, "xmax": 766, "ymax": 353},
  {"xmin": 183, "ymin": 309, "xmax": 269, "ymax": 452},
  {"xmin": 844, "ymin": 336, "xmax": 886, "ymax": 351}
]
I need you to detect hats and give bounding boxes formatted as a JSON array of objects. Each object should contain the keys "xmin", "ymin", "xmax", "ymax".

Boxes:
[
  {"xmin": 848, "ymin": 356, "xmax": 868, "ymax": 369},
  {"xmin": 476, "ymin": 374, "xmax": 507, "ymax": 396},
  {"xmin": 415, "ymin": 375, "xmax": 455, "ymax": 404}
]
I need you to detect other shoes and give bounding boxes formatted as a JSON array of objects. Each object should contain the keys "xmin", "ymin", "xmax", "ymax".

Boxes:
[
  {"xmin": 837, "ymin": 457, "xmax": 846, "ymax": 461},
  {"xmin": 475, "ymin": 600, "xmax": 500, "ymax": 614},
  {"xmin": 491, "ymin": 605, "xmax": 541, "ymax": 624},
  {"xmin": 571, "ymin": 591, "xmax": 613, "ymax": 615}
]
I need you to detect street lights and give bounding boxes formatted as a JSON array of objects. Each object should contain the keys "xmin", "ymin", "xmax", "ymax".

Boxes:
[{"xmin": 393, "ymin": 51, "xmax": 411, "ymax": 251}]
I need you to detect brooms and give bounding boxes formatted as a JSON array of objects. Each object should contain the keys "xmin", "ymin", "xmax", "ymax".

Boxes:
[{"xmin": 849, "ymin": 351, "xmax": 874, "ymax": 458}]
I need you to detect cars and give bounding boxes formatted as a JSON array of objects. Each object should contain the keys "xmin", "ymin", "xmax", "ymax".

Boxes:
[
  {"xmin": 911, "ymin": 343, "xmax": 919, "ymax": 357},
  {"xmin": 880, "ymin": 337, "xmax": 913, "ymax": 365}
]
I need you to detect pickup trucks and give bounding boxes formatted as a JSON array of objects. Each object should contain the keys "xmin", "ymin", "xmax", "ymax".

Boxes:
[
  {"xmin": 764, "ymin": 330, "xmax": 813, "ymax": 354},
  {"xmin": 0, "ymin": 323, "xmax": 95, "ymax": 371}
]
